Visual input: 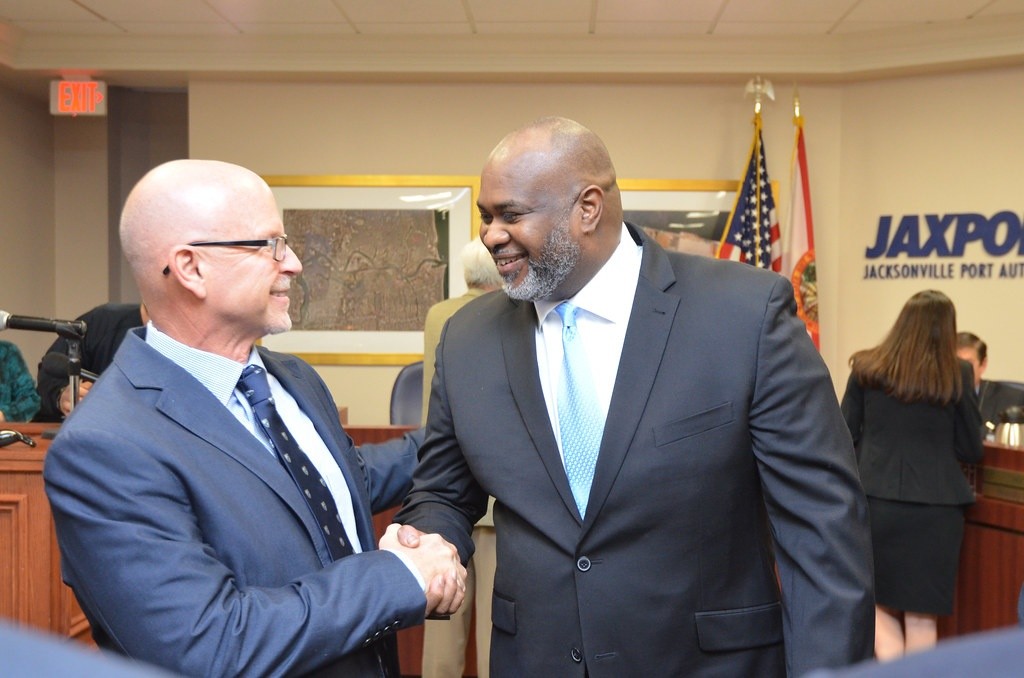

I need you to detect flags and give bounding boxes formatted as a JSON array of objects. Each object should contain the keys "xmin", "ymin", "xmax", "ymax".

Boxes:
[{"xmin": 715, "ymin": 113, "xmax": 821, "ymax": 355}]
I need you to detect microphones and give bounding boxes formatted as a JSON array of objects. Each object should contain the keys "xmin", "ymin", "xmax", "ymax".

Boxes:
[
  {"xmin": 0, "ymin": 310, "xmax": 87, "ymax": 333},
  {"xmin": 41, "ymin": 352, "xmax": 100, "ymax": 384}
]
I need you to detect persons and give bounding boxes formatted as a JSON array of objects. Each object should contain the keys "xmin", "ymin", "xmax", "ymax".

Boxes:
[
  {"xmin": 420, "ymin": 236, "xmax": 505, "ymax": 676},
  {"xmin": 38, "ymin": 299, "xmax": 152, "ymax": 424},
  {"xmin": 391, "ymin": 116, "xmax": 877, "ymax": 674},
  {"xmin": 0, "ymin": 339, "xmax": 42, "ymax": 423},
  {"xmin": 43, "ymin": 157, "xmax": 469, "ymax": 675},
  {"xmin": 841, "ymin": 289, "xmax": 1022, "ymax": 663}
]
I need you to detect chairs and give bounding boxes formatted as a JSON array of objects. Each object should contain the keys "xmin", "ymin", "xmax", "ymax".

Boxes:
[{"xmin": 390, "ymin": 361, "xmax": 424, "ymax": 425}]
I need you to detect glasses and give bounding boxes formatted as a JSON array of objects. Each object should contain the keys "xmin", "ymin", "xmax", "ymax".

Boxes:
[{"xmin": 162, "ymin": 236, "xmax": 286, "ymax": 275}]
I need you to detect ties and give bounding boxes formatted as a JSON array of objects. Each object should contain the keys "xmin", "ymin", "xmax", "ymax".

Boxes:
[
  {"xmin": 555, "ymin": 302, "xmax": 604, "ymax": 519},
  {"xmin": 237, "ymin": 363, "xmax": 354, "ymax": 563}
]
